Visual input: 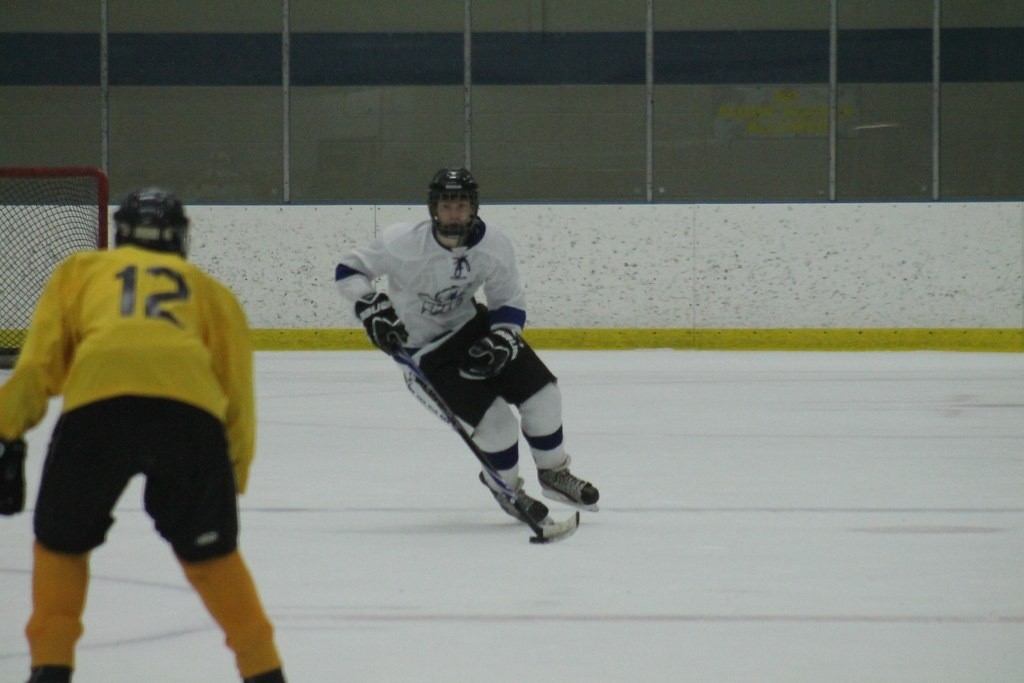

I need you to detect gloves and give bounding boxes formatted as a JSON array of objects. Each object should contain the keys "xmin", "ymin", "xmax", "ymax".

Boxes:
[
  {"xmin": 1, "ymin": 434, "xmax": 25, "ymax": 517},
  {"xmin": 460, "ymin": 326, "xmax": 525, "ymax": 382},
  {"xmin": 356, "ymin": 291, "xmax": 410, "ymax": 357}
]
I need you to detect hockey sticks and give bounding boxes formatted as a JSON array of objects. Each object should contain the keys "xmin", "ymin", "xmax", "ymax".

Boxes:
[{"xmin": 394, "ymin": 345, "xmax": 580, "ymax": 538}]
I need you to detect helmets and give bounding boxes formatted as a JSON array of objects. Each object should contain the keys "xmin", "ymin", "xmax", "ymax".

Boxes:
[
  {"xmin": 113, "ymin": 185, "xmax": 192, "ymax": 258},
  {"xmin": 427, "ymin": 167, "xmax": 482, "ymax": 238}
]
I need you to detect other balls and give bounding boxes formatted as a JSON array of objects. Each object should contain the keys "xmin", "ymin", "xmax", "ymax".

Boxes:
[{"xmin": 530, "ymin": 536, "xmax": 549, "ymax": 543}]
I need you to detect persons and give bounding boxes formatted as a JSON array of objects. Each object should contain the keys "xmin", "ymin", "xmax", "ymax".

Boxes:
[
  {"xmin": 336, "ymin": 167, "xmax": 599, "ymax": 522},
  {"xmin": 0, "ymin": 189, "xmax": 288, "ymax": 682}
]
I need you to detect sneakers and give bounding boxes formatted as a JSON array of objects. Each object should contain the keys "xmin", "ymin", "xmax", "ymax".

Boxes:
[
  {"xmin": 478, "ymin": 468, "xmax": 557, "ymax": 530},
  {"xmin": 537, "ymin": 454, "xmax": 600, "ymax": 514}
]
